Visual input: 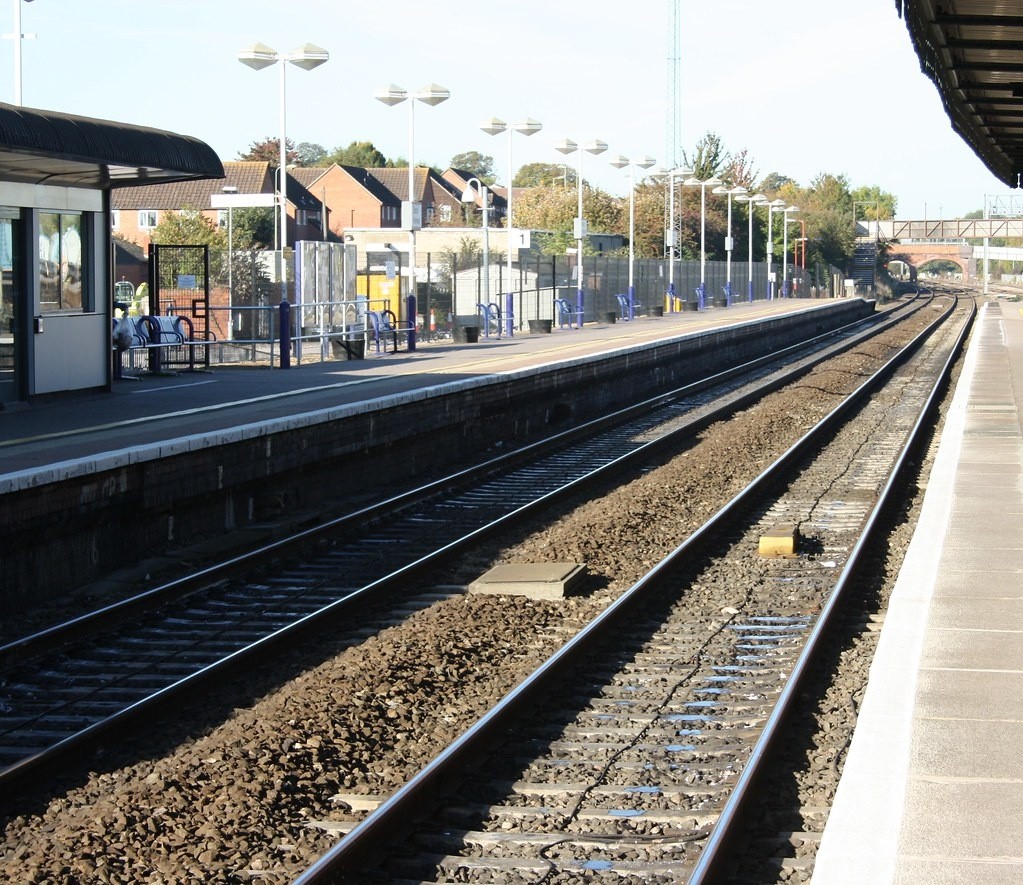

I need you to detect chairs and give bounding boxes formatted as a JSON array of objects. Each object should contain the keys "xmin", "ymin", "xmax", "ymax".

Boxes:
[
  {"xmin": 614, "ymin": 294, "xmax": 641, "ymax": 321},
  {"xmin": 476, "ymin": 302, "xmax": 513, "ymax": 340},
  {"xmin": 366, "ymin": 311, "xmax": 416, "ymax": 355},
  {"xmin": 552, "ymin": 298, "xmax": 584, "ymax": 330},
  {"xmin": 112, "ymin": 315, "xmax": 217, "ymax": 350}
]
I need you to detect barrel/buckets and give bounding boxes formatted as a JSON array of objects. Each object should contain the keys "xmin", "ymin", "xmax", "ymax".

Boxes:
[
  {"xmin": 595, "ymin": 312, "xmax": 617, "ymax": 325},
  {"xmin": 452, "ymin": 327, "xmax": 481, "ymax": 343},
  {"xmin": 712, "ymin": 298, "xmax": 727, "ymax": 307},
  {"xmin": 681, "ymin": 301, "xmax": 699, "ymax": 311},
  {"xmin": 646, "ymin": 306, "xmax": 663, "ymax": 317},
  {"xmin": 528, "ymin": 320, "xmax": 552, "ymax": 333},
  {"xmin": 332, "ymin": 340, "xmax": 366, "ymax": 360}
]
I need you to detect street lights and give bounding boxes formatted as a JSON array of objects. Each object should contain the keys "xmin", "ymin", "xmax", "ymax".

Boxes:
[
  {"xmin": 650, "ymin": 165, "xmax": 695, "ymax": 317},
  {"xmin": 481, "ymin": 116, "xmax": 543, "ymax": 339},
  {"xmin": 772, "ymin": 206, "xmax": 800, "ymax": 299},
  {"xmin": 378, "ymin": 83, "xmax": 449, "ymax": 351},
  {"xmin": 273, "ymin": 164, "xmax": 297, "ymax": 251},
  {"xmin": 557, "ymin": 138, "xmax": 608, "ymax": 328},
  {"xmin": 462, "ymin": 178, "xmax": 493, "ymax": 341},
  {"xmin": 610, "ymin": 155, "xmax": 657, "ymax": 320},
  {"xmin": 237, "ymin": 42, "xmax": 330, "ymax": 371},
  {"xmin": 710, "ymin": 185, "xmax": 748, "ymax": 308},
  {"xmin": 786, "ymin": 218, "xmax": 807, "ymax": 298},
  {"xmin": 757, "ymin": 199, "xmax": 785, "ymax": 301},
  {"xmin": 684, "ymin": 177, "xmax": 723, "ymax": 313},
  {"xmin": 735, "ymin": 194, "xmax": 767, "ymax": 304}
]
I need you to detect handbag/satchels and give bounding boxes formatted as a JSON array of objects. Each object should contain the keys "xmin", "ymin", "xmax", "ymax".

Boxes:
[{"xmin": 112, "ymin": 317, "xmax": 133, "ymax": 353}]
